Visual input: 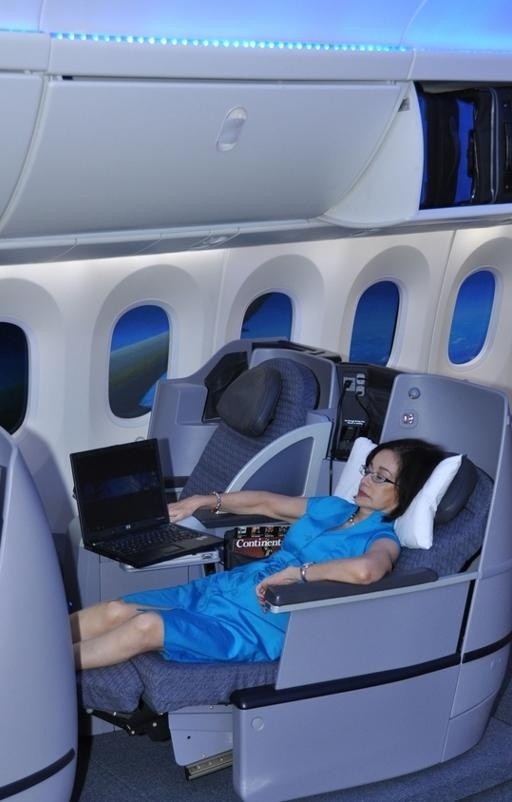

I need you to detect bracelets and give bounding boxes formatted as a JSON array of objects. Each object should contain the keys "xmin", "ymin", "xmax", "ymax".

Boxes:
[{"xmin": 209, "ymin": 489, "xmax": 225, "ymax": 519}]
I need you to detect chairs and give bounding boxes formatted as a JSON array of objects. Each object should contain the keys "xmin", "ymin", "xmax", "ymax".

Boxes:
[
  {"xmin": 79, "ymin": 361, "xmax": 512, "ymax": 802},
  {"xmin": 76, "ymin": 337, "xmax": 341, "ymax": 610},
  {"xmin": 1, "ymin": 423, "xmax": 78, "ymax": 802}
]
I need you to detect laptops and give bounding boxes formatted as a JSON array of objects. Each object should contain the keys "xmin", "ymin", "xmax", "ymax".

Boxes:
[{"xmin": 70, "ymin": 438, "xmax": 224, "ymax": 568}]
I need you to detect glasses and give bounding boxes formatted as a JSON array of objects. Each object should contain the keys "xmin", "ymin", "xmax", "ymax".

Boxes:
[{"xmin": 360, "ymin": 465, "xmax": 395, "ymax": 485}]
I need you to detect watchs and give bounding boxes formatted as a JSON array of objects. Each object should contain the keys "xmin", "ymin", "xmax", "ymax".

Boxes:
[{"xmin": 297, "ymin": 560, "xmax": 316, "ymax": 583}]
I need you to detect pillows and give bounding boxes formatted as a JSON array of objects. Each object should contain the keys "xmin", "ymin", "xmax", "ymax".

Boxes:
[{"xmin": 330, "ymin": 437, "xmax": 462, "ymax": 550}]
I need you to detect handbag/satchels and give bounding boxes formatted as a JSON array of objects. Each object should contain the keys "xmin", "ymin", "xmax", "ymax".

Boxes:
[{"xmin": 223, "ymin": 526, "xmax": 290, "ymax": 570}]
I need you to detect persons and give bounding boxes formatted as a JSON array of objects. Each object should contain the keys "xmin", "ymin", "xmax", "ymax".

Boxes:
[{"xmin": 68, "ymin": 438, "xmax": 450, "ymax": 670}]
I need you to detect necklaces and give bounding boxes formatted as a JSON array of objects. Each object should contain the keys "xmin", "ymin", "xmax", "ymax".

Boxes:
[{"xmin": 351, "ymin": 509, "xmax": 357, "ymax": 525}]
